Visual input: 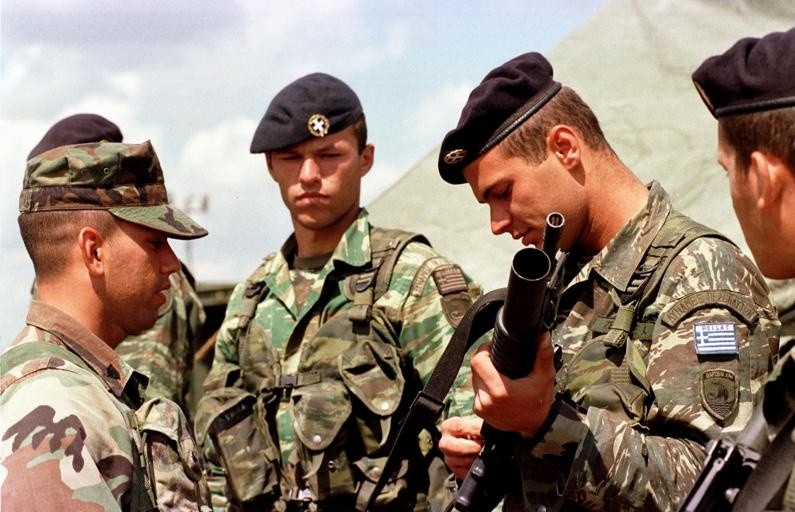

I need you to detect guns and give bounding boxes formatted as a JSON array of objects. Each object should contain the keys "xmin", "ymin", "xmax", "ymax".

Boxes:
[{"xmin": 444, "ymin": 213, "xmax": 571, "ymax": 512}]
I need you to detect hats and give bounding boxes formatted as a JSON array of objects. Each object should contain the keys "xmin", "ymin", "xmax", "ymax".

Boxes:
[
  {"xmin": 692, "ymin": 28, "xmax": 795, "ymax": 119},
  {"xmin": 439, "ymin": 53, "xmax": 561, "ymax": 184},
  {"xmin": 19, "ymin": 140, "xmax": 208, "ymax": 239},
  {"xmin": 27, "ymin": 114, "xmax": 122, "ymax": 161},
  {"xmin": 251, "ymin": 74, "xmax": 363, "ymax": 153}
]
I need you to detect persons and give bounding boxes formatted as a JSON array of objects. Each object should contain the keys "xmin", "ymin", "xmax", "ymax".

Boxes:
[
  {"xmin": 28, "ymin": 114, "xmax": 207, "ymax": 401},
  {"xmin": 0, "ymin": 139, "xmax": 209, "ymax": 512},
  {"xmin": 437, "ymin": 50, "xmax": 782, "ymax": 512},
  {"xmin": 193, "ymin": 73, "xmax": 495, "ymax": 512},
  {"xmin": 691, "ymin": 26, "xmax": 794, "ymax": 512}
]
